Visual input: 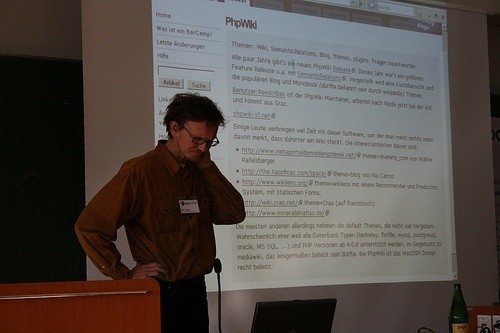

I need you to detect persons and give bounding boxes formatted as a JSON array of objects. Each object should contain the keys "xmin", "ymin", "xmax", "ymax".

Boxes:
[{"xmin": 75, "ymin": 93, "xmax": 247, "ymax": 333}]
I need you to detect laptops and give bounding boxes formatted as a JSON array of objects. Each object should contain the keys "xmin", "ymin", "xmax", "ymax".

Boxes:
[{"xmin": 251, "ymin": 298, "xmax": 337, "ymax": 333}]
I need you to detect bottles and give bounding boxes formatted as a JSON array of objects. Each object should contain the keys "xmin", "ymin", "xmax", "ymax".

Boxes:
[{"xmin": 449, "ymin": 283, "xmax": 470, "ymax": 333}]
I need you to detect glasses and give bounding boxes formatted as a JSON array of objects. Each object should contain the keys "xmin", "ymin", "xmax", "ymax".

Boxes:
[{"xmin": 178, "ymin": 121, "xmax": 220, "ymax": 147}]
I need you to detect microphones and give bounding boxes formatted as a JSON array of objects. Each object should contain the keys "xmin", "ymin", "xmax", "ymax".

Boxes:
[{"xmin": 213, "ymin": 257, "xmax": 222, "ymax": 333}]
